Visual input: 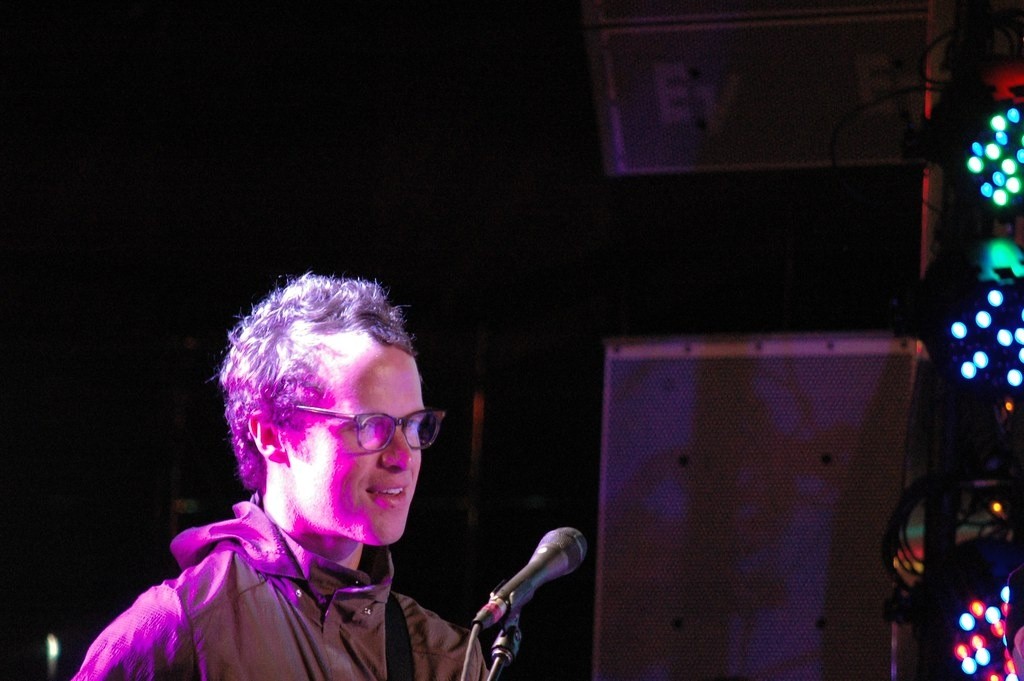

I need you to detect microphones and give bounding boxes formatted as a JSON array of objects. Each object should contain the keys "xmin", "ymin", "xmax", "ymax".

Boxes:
[{"xmin": 471, "ymin": 527, "xmax": 587, "ymax": 630}]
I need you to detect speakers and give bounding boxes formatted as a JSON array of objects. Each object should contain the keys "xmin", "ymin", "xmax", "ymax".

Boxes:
[{"xmin": 592, "ymin": 334, "xmax": 929, "ymax": 681}]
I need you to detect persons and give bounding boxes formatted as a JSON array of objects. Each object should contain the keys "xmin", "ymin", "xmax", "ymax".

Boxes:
[{"xmin": 69, "ymin": 270, "xmax": 493, "ymax": 681}]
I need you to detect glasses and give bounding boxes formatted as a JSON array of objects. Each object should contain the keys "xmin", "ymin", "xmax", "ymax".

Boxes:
[{"xmin": 287, "ymin": 405, "xmax": 446, "ymax": 453}]
